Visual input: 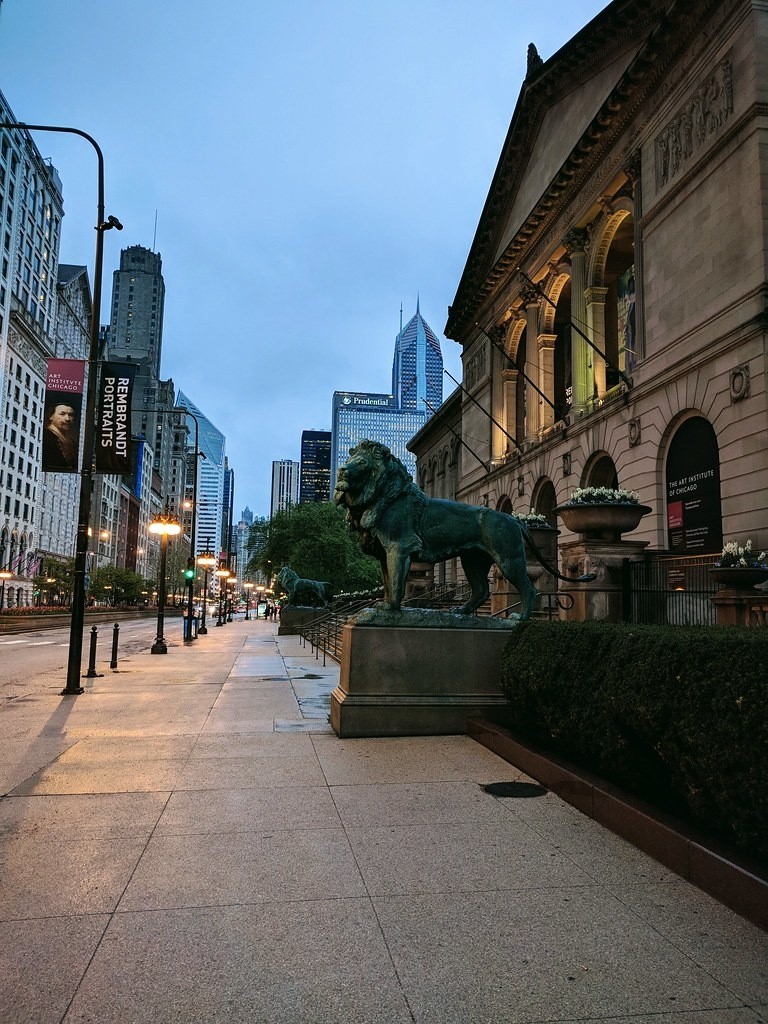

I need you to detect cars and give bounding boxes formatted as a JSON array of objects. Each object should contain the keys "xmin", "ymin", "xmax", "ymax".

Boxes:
[{"xmin": 186, "ymin": 600, "xmax": 257, "ymax": 620}]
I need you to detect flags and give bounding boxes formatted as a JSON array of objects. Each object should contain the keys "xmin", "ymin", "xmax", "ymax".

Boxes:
[
  {"xmin": 28, "ymin": 552, "xmax": 40, "ymax": 575},
  {"xmin": 14, "ymin": 550, "xmax": 25, "ymax": 568}
]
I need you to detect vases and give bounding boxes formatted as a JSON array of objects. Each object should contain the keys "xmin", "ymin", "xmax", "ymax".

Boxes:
[
  {"xmin": 552, "ymin": 503, "xmax": 652, "ymax": 540},
  {"xmin": 525, "ymin": 527, "xmax": 561, "ymax": 550},
  {"xmin": 709, "ymin": 568, "xmax": 768, "ymax": 587}
]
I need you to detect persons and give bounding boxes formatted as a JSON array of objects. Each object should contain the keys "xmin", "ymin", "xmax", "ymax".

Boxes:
[
  {"xmin": 278, "ymin": 602, "xmax": 283, "ymax": 621},
  {"xmin": 264, "ymin": 604, "xmax": 270, "ymax": 620},
  {"xmin": 44, "ymin": 403, "xmax": 77, "ymax": 469}
]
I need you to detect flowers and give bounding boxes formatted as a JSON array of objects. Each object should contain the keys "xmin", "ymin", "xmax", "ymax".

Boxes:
[
  {"xmin": 564, "ymin": 487, "xmax": 640, "ymax": 506},
  {"xmin": 334, "ymin": 586, "xmax": 385, "ymax": 600},
  {"xmin": 511, "ymin": 508, "xmax": 551, "ymax": 528},
  {"xmin": 714, "ymin": 539, "xmax": 768, "ymax": 569}
]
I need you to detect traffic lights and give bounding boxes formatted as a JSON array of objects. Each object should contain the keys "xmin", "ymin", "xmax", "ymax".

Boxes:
[{"xmin": 184, "ymin": 557, "xmax": 197, "ymax": 587}]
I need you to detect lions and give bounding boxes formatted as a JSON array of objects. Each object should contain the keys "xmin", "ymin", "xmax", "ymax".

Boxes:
[
  {"xmin": 333, "ymin": 438, "xmax": 598, "ymax": 621},
  {"xmin": 276, "ymin": 567, "xmax": 334, "ymax": 609}
]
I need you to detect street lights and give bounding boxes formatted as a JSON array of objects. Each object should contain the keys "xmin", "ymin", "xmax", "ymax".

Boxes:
[
  {"xmin": 226, "ymin": 576, "xmax": 238, "ymax": 622},
  {"xmin": 256, "ymin": 585, "xmax": 265, "ymax": 601},
  {"xmin": 147, "ymin": 510, "xmax": 187, "ymax": 655},
  {"xmin": 198, "ymin": 554, "xmax": 215, "ymax": 636},
  {"xmin": 213, "ymin": 570, "xmax": 227, "ymax": 626},
  {"xmin": 243, "ymin": 581, "xmax": 255, "ymax": 620}
]
